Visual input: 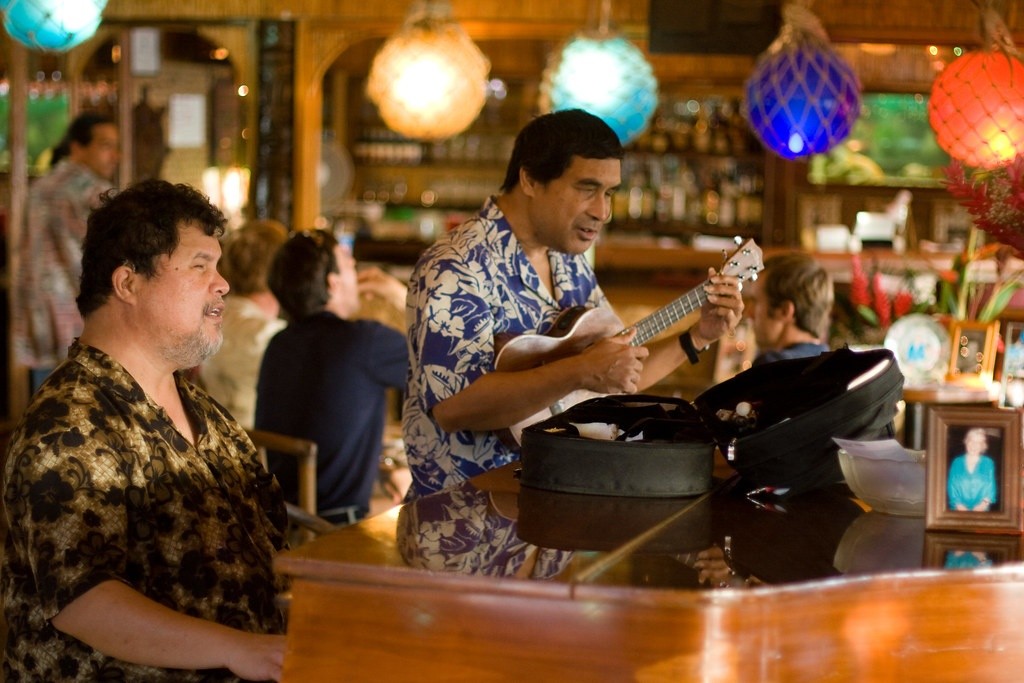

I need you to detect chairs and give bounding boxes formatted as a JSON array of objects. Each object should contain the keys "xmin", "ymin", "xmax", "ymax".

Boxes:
[{"xmin": 245, "ymin": 429, "xmax": 320, "ymax": 541}]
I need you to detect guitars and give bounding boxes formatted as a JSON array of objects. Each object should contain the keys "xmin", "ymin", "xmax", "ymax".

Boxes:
[
  {"xmin": 490, "ymin": 236, "xmax": 766, "ymax": 446},
  {"xmin": 666, "ymin": 533, "xmax": 772, "ymax": 590}
]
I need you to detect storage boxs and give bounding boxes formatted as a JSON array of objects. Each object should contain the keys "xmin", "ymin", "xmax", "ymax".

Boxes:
[
  {"xmin": 854, "ymin": 209, "xmax": 894, "ymax": 249},
  {"xmin": 930, "ymin": 193, "xmax": 986, "ymax": 250}
]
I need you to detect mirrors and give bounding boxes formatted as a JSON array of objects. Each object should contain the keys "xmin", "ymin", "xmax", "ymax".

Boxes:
[{"xmin": 116, "ymin": 20, "xmax": 295, "ymax": 235}]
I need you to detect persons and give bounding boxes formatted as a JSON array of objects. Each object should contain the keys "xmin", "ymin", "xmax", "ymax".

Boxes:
[
  {"xmin": 2, "ymin": 112, "xmax": 127, "ymax": 401},
  {"xmin": 252, "ymin": 225, "xmax": 410, "ymax": 547},
  {"xmin": 0, "ymin": 173, "xmax": 304, "ymax": 682},
  {"xmin": 745, "ymin": 251, "xmax": 836, "ymax": 378},
  {"xmin": 386, "ymin": 107, "xmax": 745, "ymax": 508},
  {"xmin": 944, "ymin": 427, "xmax": 999, "ymax": 514}
]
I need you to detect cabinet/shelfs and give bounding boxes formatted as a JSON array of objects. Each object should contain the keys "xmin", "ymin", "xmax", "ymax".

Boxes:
[{"xmin": 331, "ymin": 51, "xmax": 969, "ymax": 279}]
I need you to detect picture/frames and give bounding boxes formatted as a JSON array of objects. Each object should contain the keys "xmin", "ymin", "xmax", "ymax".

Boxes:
[
  {"xmin": 993, "ymin": 322, "xmax": 1024, "ymax": 411},
  {"xmin": 947, "ymin": 319, "xmax": 999, "ymax": 392},
  {"xmin": 922, "ymin": 532, "xmax": 1019, "ymax": 570},
  {"xmin": 924, "ymin": 403, "xmax": 1024, "ymax": 537}
]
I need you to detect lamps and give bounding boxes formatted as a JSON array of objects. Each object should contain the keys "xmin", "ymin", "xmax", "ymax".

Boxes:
[
  {"xmin": 746, "ymin": 0, "xmax": 861, "ymax": 161},
  {"xmin": 361, "ymin": 0, "xmax": 497, "ymax": 147},
  {"xmin": 0, "ymin": 0, "xmax": 109, "ymax": 56},
  {"xmin": 539, "ymin": 0, "xmax": 662, "ymax": 146},
  {"xmin": 924, "ymin": 0, "xmax": 1024, "ymax": 174}
]
